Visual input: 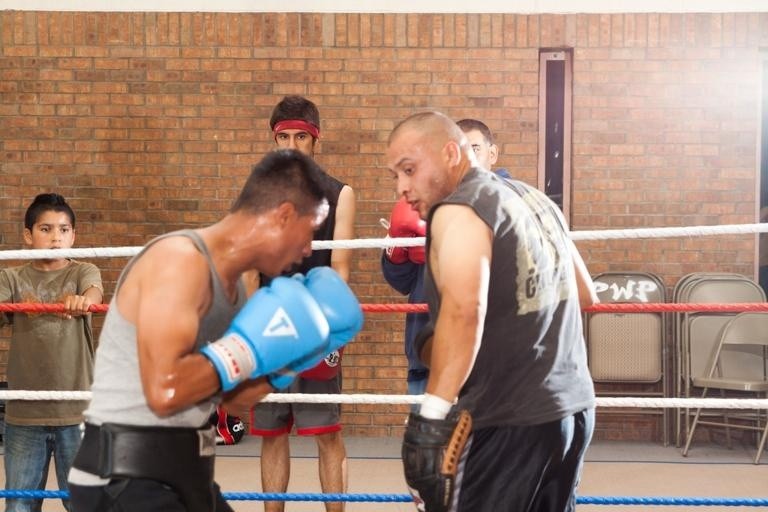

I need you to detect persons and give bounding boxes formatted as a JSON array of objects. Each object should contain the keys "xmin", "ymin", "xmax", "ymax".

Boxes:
[
  {"xmin": 237, "ymin": 95, "xmax": 360, "ymax": 512},
  {"xmin": 65, "ymin": 148, "xmax": 365, "ymax": 511},
  {"xmin": 0, "ymin": 191, "xmax": 109, "ymax": 512},
  {"xmin": 385, "ymin": 111, "xmax": 606, "ymax": 512},
  {"xmin": 379, "ymin": 118, "xmax": 511, "ymax": 512}
]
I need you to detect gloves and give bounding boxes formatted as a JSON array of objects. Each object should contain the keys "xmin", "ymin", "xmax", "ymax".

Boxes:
[
  {"xmin": 198, "ymin": 266, "xmax": 364, "ymax": 393},
  {"xmin": 385, "ymin": 198, "xmax": 428, "ymax": 265},
  {"xmin": 402, "ymin": 406, "xmax": 473, "ymax": 512}
]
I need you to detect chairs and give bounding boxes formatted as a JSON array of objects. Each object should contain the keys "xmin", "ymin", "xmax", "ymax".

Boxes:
[
  {"xmin": 672, "ymin": 272, "xmax": 768, "ymax": 465},
  {"xmin": 580, "ymin": 271, "xmax": 668, "ymax": 448}
]
